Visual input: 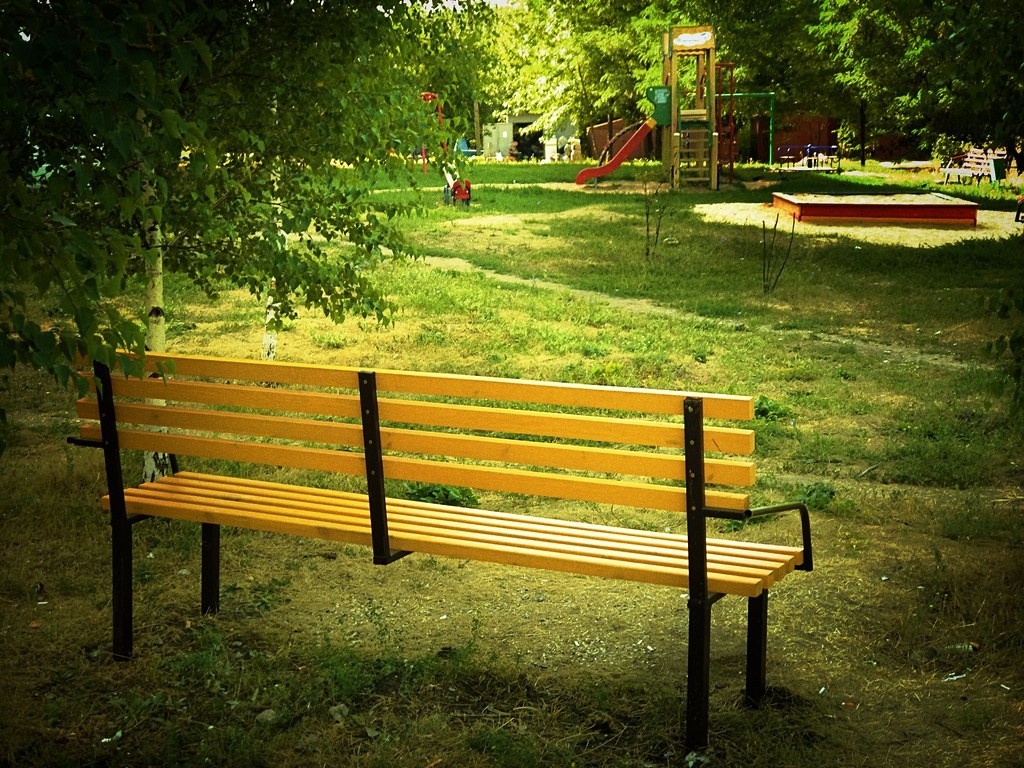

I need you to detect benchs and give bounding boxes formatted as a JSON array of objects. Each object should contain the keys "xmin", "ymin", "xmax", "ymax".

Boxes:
[
  {"xmin": 940, "ymin": 147, "xmax": 1011, "ymax": 188},
  {"xmin": 66, "ymin": 348, "xmax": 815, "ymax": 760}
]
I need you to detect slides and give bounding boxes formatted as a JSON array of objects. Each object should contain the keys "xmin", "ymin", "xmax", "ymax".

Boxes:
[{"xmin": 575, "ymin": 109, "xmax": 660, "ymax": 186}]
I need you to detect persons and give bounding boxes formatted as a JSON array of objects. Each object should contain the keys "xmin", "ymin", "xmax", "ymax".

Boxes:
[{"xmin": 507, "ymin": 141, "xmax": 524, "ymax": 162}]
